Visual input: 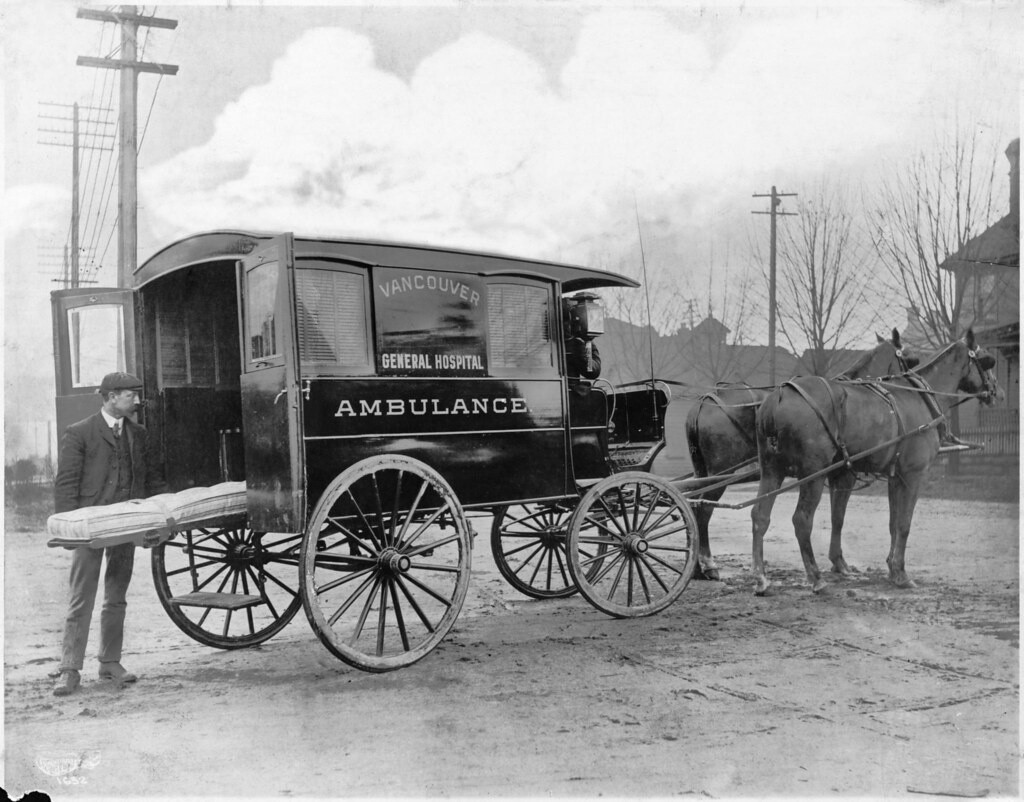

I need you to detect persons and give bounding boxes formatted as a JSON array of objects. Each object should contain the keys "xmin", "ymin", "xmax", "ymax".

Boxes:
[
  {"xmin": 558, "ymin": 296, "xmax": 615, "ymax": 464},
  {"xmin": 50, "ymin": 370, "xmax": 151, "ymax": 698}
]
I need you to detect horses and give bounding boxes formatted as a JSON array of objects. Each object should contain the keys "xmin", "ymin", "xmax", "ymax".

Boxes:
[
  {"xmin": 685, "ymin": 326, "xmax": 920, "ymax": 581},
  {"xmin": 751, "ymin": 329, "xmax": 1007, "ymax": 597}
]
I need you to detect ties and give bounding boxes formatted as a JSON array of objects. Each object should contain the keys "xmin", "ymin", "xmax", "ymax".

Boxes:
[{"xmin": 112, "ymin": 423, "xmax": 121, "ymax": 440}]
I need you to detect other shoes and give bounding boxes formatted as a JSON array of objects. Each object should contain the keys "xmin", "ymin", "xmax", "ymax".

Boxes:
[
  {"xmin": 54, "ymin": 672, "xmax": 81, "ymax": 696},
  {"xmin": 98, "ymin": 662, "xmax": 134, "ymax": 682}
]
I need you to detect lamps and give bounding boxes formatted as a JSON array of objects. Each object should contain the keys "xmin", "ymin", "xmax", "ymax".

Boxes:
[{"xmin": 570, "ymin": 291, "xmax": 604, "ymax": 343}]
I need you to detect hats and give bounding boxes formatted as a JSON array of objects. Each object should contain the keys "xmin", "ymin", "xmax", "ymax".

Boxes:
[{"xmin": 101, "ymin": 372, "xmax": 143, "ymax": 394}]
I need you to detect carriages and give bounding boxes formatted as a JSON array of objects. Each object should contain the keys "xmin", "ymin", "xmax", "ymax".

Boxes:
[{"xmin": 44, "ymin": 227, "xmax": 1006, "ymax": 671}]
